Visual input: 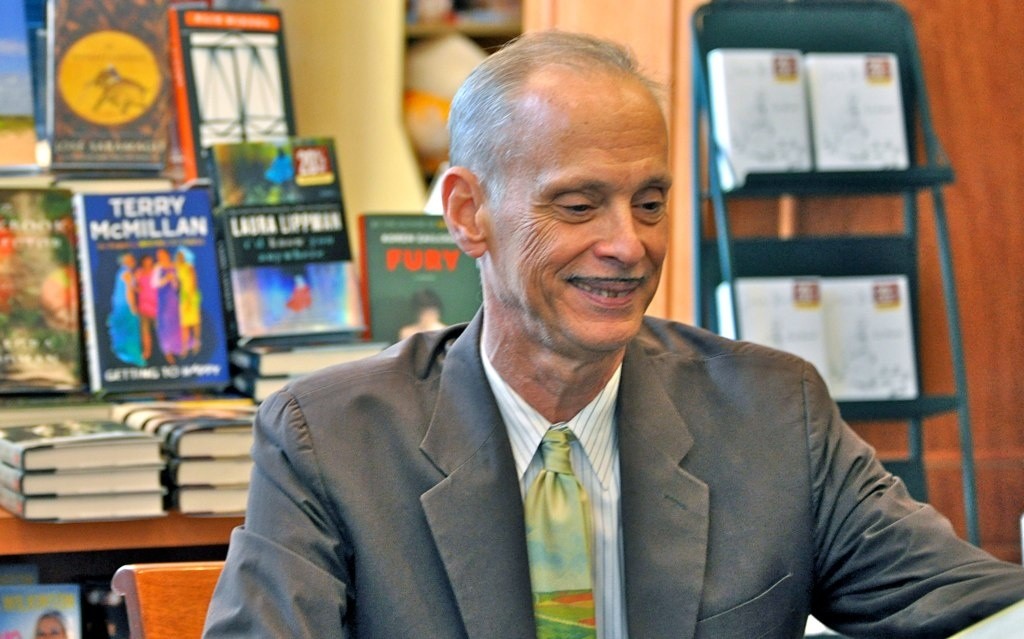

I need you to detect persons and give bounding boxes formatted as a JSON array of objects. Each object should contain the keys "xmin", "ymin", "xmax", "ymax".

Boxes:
[{"xmin": 201, "ymin": 30, "xmax": 1024, "ymax": 639}]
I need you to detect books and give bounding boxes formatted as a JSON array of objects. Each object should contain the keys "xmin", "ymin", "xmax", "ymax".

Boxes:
[{"xmin": 1, "ymin": 1, "xmax": 484, "ymax": 639}]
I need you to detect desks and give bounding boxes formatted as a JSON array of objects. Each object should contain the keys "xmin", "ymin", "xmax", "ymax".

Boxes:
[{"xmin": 0, "ymin": 506, "xmax": 244, "ymax": 558}]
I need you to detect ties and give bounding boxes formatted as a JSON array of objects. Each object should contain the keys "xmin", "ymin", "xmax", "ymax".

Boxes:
[{"xmin": 524, "ymin": 428, "xmax": 598, "ymax": 639}]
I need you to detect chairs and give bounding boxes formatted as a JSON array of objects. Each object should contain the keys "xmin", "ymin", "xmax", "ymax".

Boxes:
[{"xmin": 110, "ymin": 561, "xmax": 225, "ymax": 639}]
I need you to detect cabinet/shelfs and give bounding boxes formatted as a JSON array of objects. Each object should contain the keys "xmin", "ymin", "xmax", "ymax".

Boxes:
[{"xmin": 690, "ymin": 0, "xmax": 984, "ymax": 546}]
